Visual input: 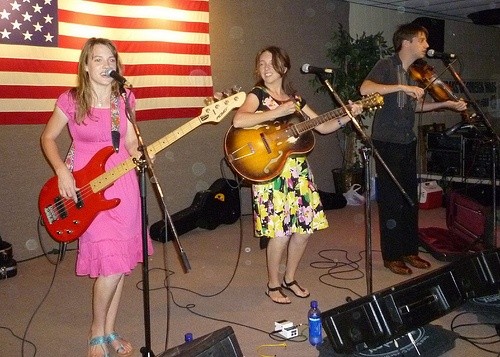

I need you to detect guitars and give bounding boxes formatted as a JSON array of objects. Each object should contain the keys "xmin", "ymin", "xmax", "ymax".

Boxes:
[
  {"xmin": 38, "ymin": 84, "xmax": 247, "ymax": 243},
  {"xmin": 223, "ymin": 91, "xmax": 385, "ymax": 184}
]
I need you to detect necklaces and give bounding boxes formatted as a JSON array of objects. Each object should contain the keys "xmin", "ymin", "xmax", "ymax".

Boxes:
[{"xmin": 96, "ymin": 93, "xmax": 109, "ymax": 107}]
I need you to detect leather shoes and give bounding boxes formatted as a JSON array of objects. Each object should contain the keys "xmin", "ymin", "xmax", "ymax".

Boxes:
[
  {"xmin": 404, "ymin": 254, "xmax": 431, "ymax": 269},
  {"xmin": 384, "ymin": 260, "xmax": 414, "ymax": 275}
]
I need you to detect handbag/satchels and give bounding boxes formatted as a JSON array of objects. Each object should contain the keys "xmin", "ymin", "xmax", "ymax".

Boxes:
[{"xmin": 343, "ymin": 184, "xmax": 366, "ymax": 206}]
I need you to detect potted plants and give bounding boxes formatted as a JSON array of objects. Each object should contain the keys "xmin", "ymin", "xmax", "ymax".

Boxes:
[{"xmin": 312, "ymin": 22, "xmax": 390, "ymax": 195}]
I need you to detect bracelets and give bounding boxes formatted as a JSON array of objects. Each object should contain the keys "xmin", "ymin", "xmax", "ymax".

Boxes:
[{"xmin": 337, "ymin": 117, "xmax": 345, "ymax": 127}]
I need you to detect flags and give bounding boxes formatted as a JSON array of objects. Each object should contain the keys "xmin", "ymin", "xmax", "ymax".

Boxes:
[{"xmin": 0, "ymin": 0, "xmax": 211, "ymax": 125}]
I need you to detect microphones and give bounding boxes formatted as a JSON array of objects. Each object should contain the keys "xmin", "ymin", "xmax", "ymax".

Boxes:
[
  {"xmin": 443, "ymin": 113, "xmax": 477, "ymax": 139},
  {"xmin": 302, "ymin": 63, "xmax": 333, "ymax": 75},
  {"xmin": 106, "ymin": 69, "xmax": 133, "ymax": 88},
  {"xmin": 427, "ymin": 49, "xmax": 455, "ymax": 60}
]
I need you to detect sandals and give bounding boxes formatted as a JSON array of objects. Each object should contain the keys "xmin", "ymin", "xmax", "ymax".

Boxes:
[
  {"xmin": 281, "ymin": 276, "xmax": 310, "ymax": 298},
  {"xmin": 265, "ymin": 283, "xmax": 291, "ymax": 304}
]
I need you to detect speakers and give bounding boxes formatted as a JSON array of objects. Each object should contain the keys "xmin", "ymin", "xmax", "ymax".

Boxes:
[
  {"xmin": 321, "ymin": 261, "xmax": 469, "ymax": 348},
  {"xmin": 154, "ymin": 325, "xmax": 243, "ymax": 357},
  {"xmin": 454, "ymin": 245, "xmax": 500, "ymax": 299},
  {"xmin": 425, "ymin": 131, "xmax": 500, "ymax": 178}
]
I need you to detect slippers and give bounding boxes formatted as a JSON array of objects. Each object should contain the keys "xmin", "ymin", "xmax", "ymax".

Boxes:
[
  {"xmin": 105, "ymin": 331, "xmax": 136, "ymax": 357},
  {"xmin": 87, "ymin": 331, "xmax": 109, "ymax": 357}
]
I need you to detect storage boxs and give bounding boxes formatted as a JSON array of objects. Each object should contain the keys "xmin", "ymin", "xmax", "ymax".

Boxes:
[{"xmin": 418, "ymin": 182, "xmax": 443, "ymax": 209}]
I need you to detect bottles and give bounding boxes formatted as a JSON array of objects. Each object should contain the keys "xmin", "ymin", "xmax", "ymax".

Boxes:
[
  {"xmin": 184, "ymin": 333, "xmax": 194, "ymax": 343},
  {"xmin": 308, "ymin": 301, "xmax": 322, "ymax": 346}
]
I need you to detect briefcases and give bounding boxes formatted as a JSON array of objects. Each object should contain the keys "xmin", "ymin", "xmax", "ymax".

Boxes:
[
  {"xmin": 0, "ymin": 241, "xmax": 17, "ymax": 280},
  {"xmin": 418, "ymin": 190, "xmax": 493, "ymax": 262}
]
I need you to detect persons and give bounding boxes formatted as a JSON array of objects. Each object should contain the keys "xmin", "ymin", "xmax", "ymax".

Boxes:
[
  {"xmin": 360, "ymin": 23, "xmax": 468, "ymax": 276},
  {"xmin": 40, "ymin": 38, "xmax": 157, "ymax": 357},
  {"xmin": 233, "ymin": 47, "xmax": 364, "ymax": 303}
]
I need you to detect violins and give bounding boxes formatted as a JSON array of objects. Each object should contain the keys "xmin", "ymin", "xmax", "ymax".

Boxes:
[{"xmin": 409, "ymin": 62, "xmax": 481, "ymax": 124}]
